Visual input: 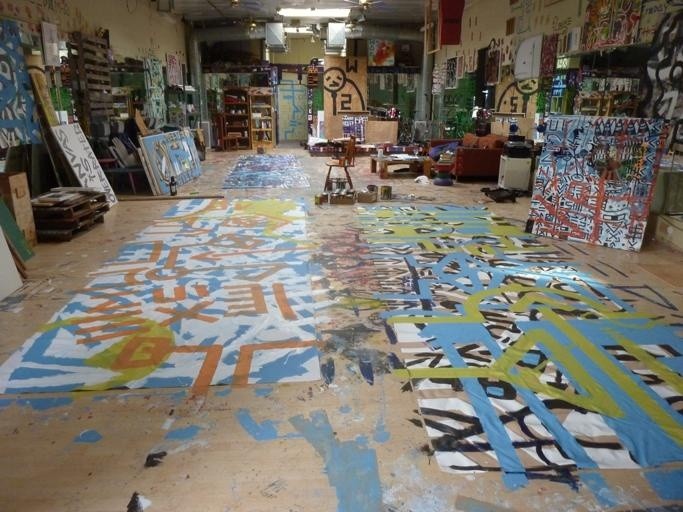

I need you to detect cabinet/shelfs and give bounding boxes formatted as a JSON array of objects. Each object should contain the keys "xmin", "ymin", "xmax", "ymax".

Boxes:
[
  {"xmin": 222, "ymin": 89, "xmax": 275, "ymax": 151},
  {"xmin": 497, "ymin": 154, "xmax": 532, "ymax": 192}
]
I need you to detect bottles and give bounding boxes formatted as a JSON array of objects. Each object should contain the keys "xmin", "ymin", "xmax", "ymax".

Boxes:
[{"xmin": 169, "ymin": 176, "xmax": 177, "ymax": 196}]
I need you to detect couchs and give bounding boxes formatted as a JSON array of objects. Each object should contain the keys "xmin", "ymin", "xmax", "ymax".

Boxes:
[{"xmin": 428, "ymin": 133, "xmax": 504, "ymax": 183}]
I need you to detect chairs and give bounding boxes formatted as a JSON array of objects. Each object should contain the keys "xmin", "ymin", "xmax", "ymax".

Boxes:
[{"xmin": 323, "ymin": 135, "xmax": 355, "ymax": 193}]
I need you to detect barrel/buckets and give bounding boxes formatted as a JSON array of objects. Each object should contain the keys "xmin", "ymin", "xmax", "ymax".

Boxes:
[
  {"xmin": 327, "ymin": 178, "xmax": 346, "ymax": 191},
  {"xmin": 381, "ymin": 185, "xmax": 392, "ymax": 200}
]
